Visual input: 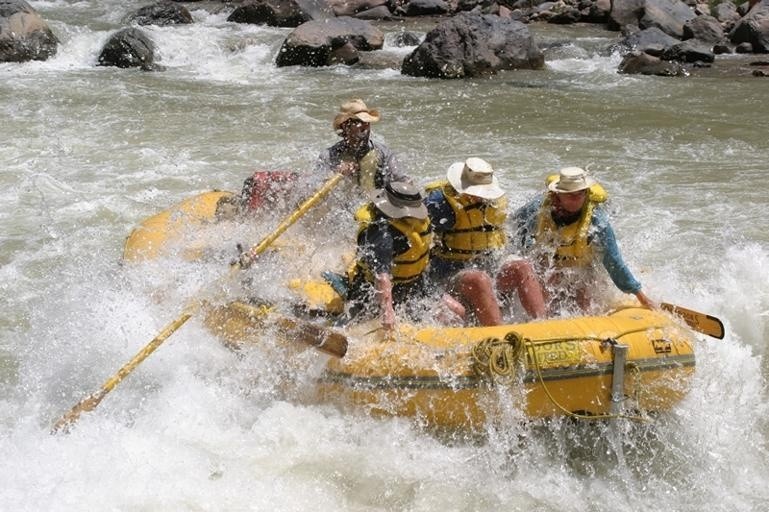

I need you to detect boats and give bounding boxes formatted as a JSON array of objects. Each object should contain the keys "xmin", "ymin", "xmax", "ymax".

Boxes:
[{"xmin": 120, "ymin": 190, "xmax": 697, "ymax": 435}]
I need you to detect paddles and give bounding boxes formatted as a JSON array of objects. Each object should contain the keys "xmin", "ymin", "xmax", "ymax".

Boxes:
[
  {"xmin": 49, "ymin": 172, "xmax": 346, "ymax": 435},
  {"xmin": 661, "ymin": 301, "xmax": 724, "ymax": 340},
  {"xmin": 229, "ymin": 303, "xmax": 349, "ymax": 357}
]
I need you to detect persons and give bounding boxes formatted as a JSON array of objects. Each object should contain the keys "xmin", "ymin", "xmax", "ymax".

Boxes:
[
  {"xmin": 506, "ymin": 167, "xmax": 660, "ymax": 317},
  {"xmin": 305, "ymin": 98, "xmax": 412, "ymax": 242},
  {"xmin": 337, "ymin": 181, "xmax": 465, "ymax": 331},
  {"xmin": 421, "ymin": 157, "xmax": 547, "ymax": 327}
]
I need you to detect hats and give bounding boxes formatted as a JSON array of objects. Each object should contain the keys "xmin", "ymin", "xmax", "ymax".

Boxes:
[
  {"xmin": 333, "ymin": 97, "xmax": 380, "ymax": 132},
  {"xmin": 370, "ymin": 181, "xmax": 429, "ymax": 219},
  {"xmin": 446, "ymin": 157, "xmax": 506, "ymax": 199},
  {"xmin": 548, "ymin": 166, "xmax": 596, "ymax": 193}
]
